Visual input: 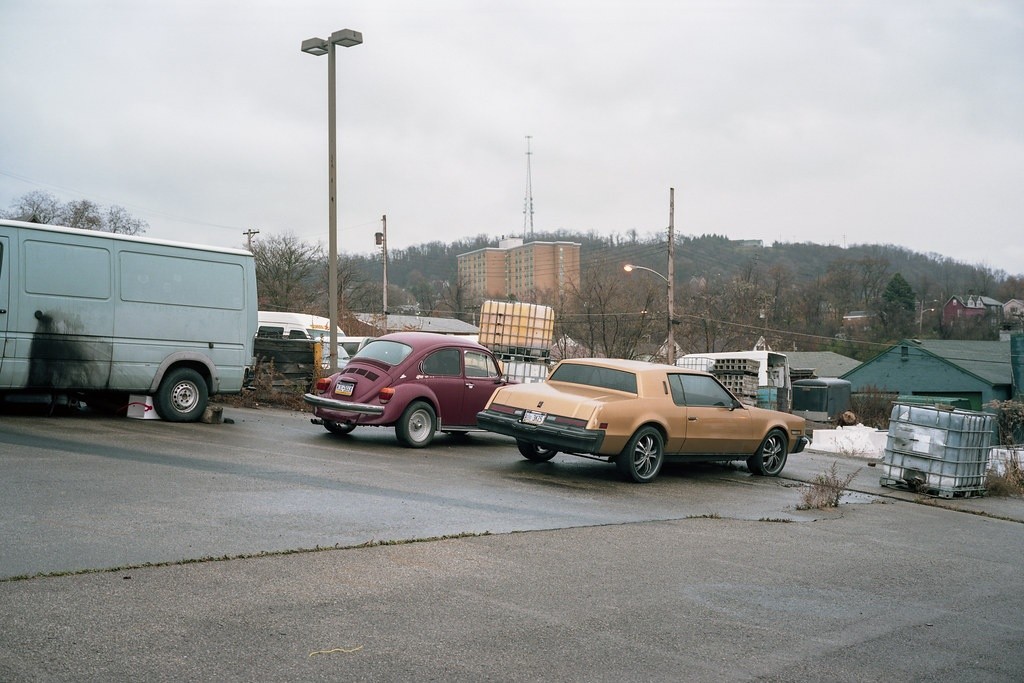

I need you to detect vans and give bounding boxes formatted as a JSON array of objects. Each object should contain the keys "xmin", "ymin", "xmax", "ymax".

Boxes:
[
  {"xmin": 449, "ymin": 334, "xmax": 548, "ymax": 384},
  {"xmin": 0, "ymin": 219, "xmax": 259, "ymax": 422},
  {"xmin": 672, "ymin": 352, "xmax": 793, "ymax": 412},
  {"xmin": 315, "ymin": 336, "xmax": 374, "ymax": 370},
  {"xmin": 256, "ymin": 310, "xmax": 344, "ymax": 340}
]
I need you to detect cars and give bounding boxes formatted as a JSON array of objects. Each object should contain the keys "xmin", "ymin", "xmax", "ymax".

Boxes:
[
  {"xmin": 303, "ymin": 332, "xmax": 521, "ymax": 449},
  {"xmin": 476, "ymin": 353, "xmax": 814, "ymax": 483},
  {"xmin": 320, "ymin": 343, "xmax": 350, "ymax": 369}
]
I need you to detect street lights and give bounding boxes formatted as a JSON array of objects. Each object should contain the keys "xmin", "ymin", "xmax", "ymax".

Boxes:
[
  {"xmin": 301, "ymin": 29, "xmax": 364, "ymax": 374},
  {"xmin": 624, "ymin": 264, "xmax": 675, "ymax": 366},
  {"xmin": 919, "ymin": 309, "xmax": 935, "ymax": 337}
]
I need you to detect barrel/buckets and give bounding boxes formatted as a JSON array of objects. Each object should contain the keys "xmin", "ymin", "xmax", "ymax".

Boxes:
[{"xmin": 756, "ymin": 386, "xmax": 790, "ymax": 414}]
